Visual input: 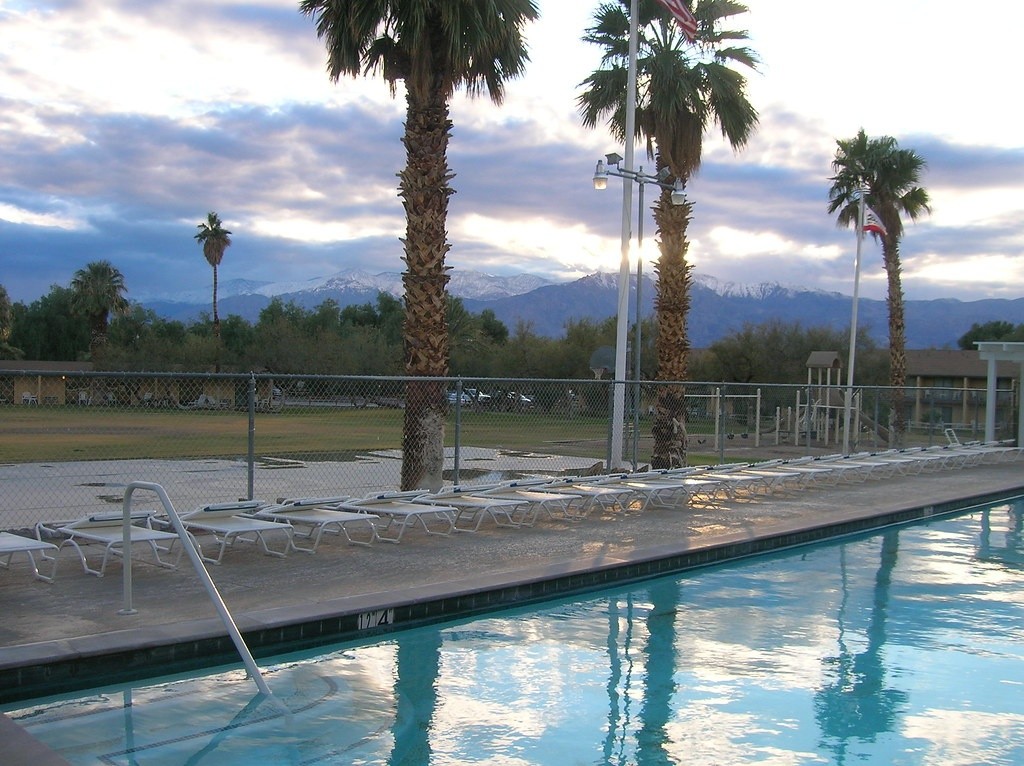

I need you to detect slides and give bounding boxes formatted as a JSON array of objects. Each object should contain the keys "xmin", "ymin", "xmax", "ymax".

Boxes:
[
  {"xmin": 760, "ymin": 404, "xmax": 807, "ymax": 436},
  {"xmin": 853, "ymin": 409, "xmax": 889, "ymax": 446}
]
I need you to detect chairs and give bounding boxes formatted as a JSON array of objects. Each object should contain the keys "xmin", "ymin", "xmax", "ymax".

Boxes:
[
  {"xmin": 22, "ymin": 392, "xmax": 38, "ymax": 404},
  {"xmin": 79, "ymin": 391, "xmax": 87, "ymax": 406},
  {"xmin": 106, "ymin": 392, "xmax": 116, "ymax": 406},
  {"xmin": 1, "ymin": 428, "xmax": 1024, "ymax": 585}
]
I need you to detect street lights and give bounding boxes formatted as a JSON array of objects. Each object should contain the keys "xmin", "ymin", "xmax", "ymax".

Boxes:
[
  {"xmin": 842, "ymin": 181, "xmax": 871, "ymax": 457},
  {"xmin": 591, "ymin": 154, "xmax": 686, "ymax": 476}
]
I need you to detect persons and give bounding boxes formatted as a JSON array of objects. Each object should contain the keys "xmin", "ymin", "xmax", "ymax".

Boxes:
[
  {"xmin": 159, "ymin": 390, "xmax": 176, "ymax": 409},
  {"xmin": 147, "ymin": 394, "xmax": 158, "ymax": 409},
  {"xmin": 134, "ymin": 387, "xmax": 147, "ymax": 401}
]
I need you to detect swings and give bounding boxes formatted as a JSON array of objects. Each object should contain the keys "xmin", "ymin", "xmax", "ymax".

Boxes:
[
  {"xmin": 698, "ymin": 397, "xmax": 706, "ymax": 444},
  {"xmin": 726, "ymin": 397, "xmax": 735, "ymax": 440},
  {"xmin": 741, "ymin": 397, "xmax": 748, "ymax": 440}
]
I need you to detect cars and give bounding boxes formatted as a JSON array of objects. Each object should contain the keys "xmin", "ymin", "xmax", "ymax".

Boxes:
[
  {"xmin": 448, "ymin": 388, "xmax": 536, "ymax": 406},
  {"xmin": 371, "ymin": 396, "xmax": 405, "ymax": 408}
]
